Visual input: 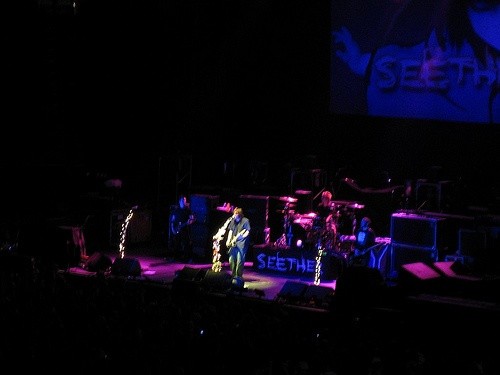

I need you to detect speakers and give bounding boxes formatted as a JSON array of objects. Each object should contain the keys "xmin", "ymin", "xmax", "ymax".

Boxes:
[
  {"xmin": 336, "ymin": 257, "xmax": 378, "ymax": 306},
  {"xmin": 238, "ymin": 194, "xmax": 275, "ymax": 244},
  {"xmin": 278, "ymin": 280, "xmax": 310, "ymax": 303},
  {"xmin": 57, "ymin": 226, "xmax": 86, "ymax": 266},
  {"xmin": 86, "ymin": 252, "xmax": 111, "ymax": 272},
  {"xmin": 177, "ymin": 266, "xmax": 207, "ymax": 282},
  {"xmin": 391, "ymin": 212, "xmax": 458, "ymax": 248},
  {"xmin": 390, "ymin": 242, "xmax": 456, "ymax": 282},
  {"xmin": 112, "ymin": 257, "xmax": 141, "ymax": 276},
  {"xmin": 189, "ymin": 194, "xmax": 218, "ymax": 226}
]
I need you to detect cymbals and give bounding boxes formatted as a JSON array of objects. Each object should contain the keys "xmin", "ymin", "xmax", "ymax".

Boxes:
[
  {"xmin": 295, "ymin": 190, "xmax": 312, "ymax": 195},
  {"xmin": 279, "ymin": 196, "xmax": 298, "ymax": 202},
  {"xmin": 347, "ymin": 205, "xmax": 365, "ymax": 209}
]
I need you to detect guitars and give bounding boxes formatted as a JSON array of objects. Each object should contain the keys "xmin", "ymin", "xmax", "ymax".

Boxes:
[
  {"xmin": 224, "ymin": 234, "xmax": 243, "ymax": 254},
  {"xmin": 172, "ymin": 218, "xmax": 195, "ymax": 235}
]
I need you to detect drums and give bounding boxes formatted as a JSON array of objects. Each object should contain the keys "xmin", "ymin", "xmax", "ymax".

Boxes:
[{"xmin": 292, "ymin": 212, "xmax": 336, "ymax": 240}]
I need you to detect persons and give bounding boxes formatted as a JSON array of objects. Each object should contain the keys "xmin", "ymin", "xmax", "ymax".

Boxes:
[
  {"xmin": 352, "ymin": 216, "xmax": 378, "ymax": 264},
  {"xmin": 171, "ymin": 196, "xmax": 196, "ymax": 261},
  {"xmin": 226, "ymin": 208, "xmax": 251, "ymax": 284},
  {"xmin": 316, "ymin": 190, "xmax": 342, "ymax": 245}
]
